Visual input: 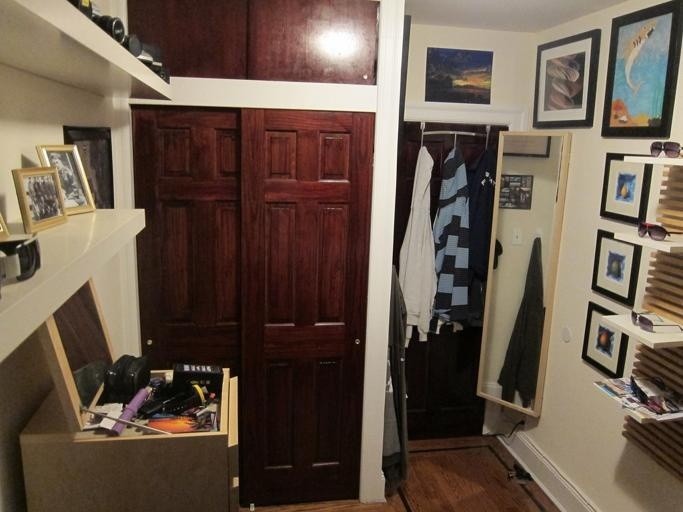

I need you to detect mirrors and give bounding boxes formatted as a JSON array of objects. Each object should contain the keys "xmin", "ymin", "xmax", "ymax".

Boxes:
[
  {"xmin": 37, "ymin": 279, "xmax": 113, "ymax": 433},
  {"xmin": 476, "ymin": 131, "xmax": 573, "ymax": 419}
]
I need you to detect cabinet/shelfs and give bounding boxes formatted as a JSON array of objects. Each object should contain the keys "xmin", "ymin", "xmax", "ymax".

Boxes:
[
  {"xmin": 0, "ymin": 0, "xmax": 172, "ymax": 367},
  {"xmin": 20, "ymin": 367, "xmax": 239, "ymax": 512},
  {"xmin": 593, "ymin": 156, "xmax": 683, "ymax": 427}
]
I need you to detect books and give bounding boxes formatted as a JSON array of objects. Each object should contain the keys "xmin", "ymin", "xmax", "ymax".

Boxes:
[{"xmin": 594, "ymin": 376, "xmax": 683, "ymax": 425}]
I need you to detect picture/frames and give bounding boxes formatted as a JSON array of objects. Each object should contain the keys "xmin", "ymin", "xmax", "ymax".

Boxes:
[
  {"xmin": 601, "ymin": 0, "xmax": 683, "ymax": 137},
  {"xmin": 36, "ymin": 145, "xmax": 96, "ymax": 216},
  {"xmin": 0, "ymin": 212, "xmax": 10, "ymax": 240},
  {"xmin": 63, "ymin": 125, "xmax": 114, "ymax": 209},
  {"xmin": 533, "ymin": 29, "xmax": 601, "ymax": 127},
  {"xmin": 582, "ymin": 152, "xmax": 655, "ymax": 379},
  {"xmin": 12, "ymin": 167, "xmax": 67, "ymax": 234}
]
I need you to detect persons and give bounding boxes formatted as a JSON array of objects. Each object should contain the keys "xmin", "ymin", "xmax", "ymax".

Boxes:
[
  {"xmin": 26, "ymin": 176, "xmax": 58, "ymax": 218},
  {"xmin": 50, "ymin": 152, "xmax": 79, "ymax": 200}
]
